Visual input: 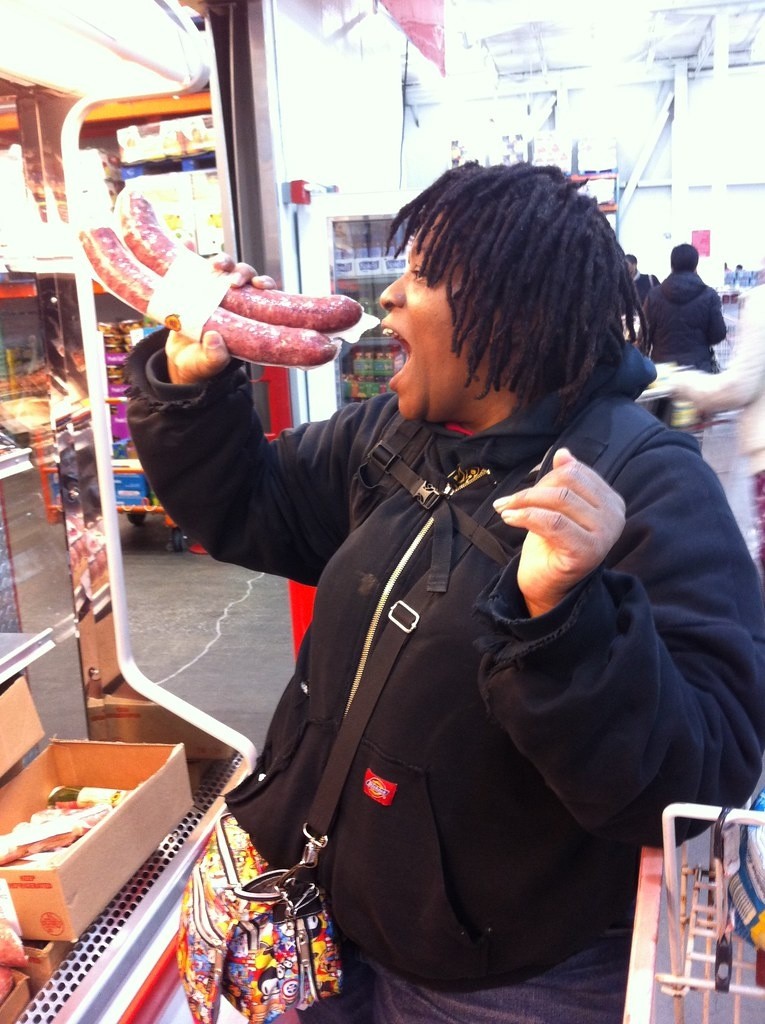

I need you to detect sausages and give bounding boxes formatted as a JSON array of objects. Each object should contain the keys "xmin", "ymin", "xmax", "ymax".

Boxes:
[{"xmin": 77, "ymin": 191, "xmax": 365, "ymax": 368}]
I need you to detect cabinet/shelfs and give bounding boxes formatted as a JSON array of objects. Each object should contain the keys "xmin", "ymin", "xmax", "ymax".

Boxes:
[{"xmin": 327, "ymin": 210, "xmax": 416, "ymax": 411}]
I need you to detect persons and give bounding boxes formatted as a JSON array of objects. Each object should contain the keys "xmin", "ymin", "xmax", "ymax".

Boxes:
[
  {"xmin": 625, "ymin": 254, "xmax": 660, "ymax": 308},
  {"xmin": 124, "ymin": 161, "xmax": 764, "ymax": 1024},
  {"xmin": 637, "ymin": 243, "xmax": 727, "ymax": 375},
  {"xmin": 673, "ymin": 281, "xmax": 764, "ymax": 566}
]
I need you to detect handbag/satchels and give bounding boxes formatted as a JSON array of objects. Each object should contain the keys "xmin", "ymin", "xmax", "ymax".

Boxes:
[{"xmin": 177, "ymin": 809, "xmax": 344, "ymax": 1023}]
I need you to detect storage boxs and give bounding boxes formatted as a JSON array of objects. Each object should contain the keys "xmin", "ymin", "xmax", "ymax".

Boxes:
[{"xmin": 0, "ymin": 679, "xmax": 187, "ymax": 1024}]
